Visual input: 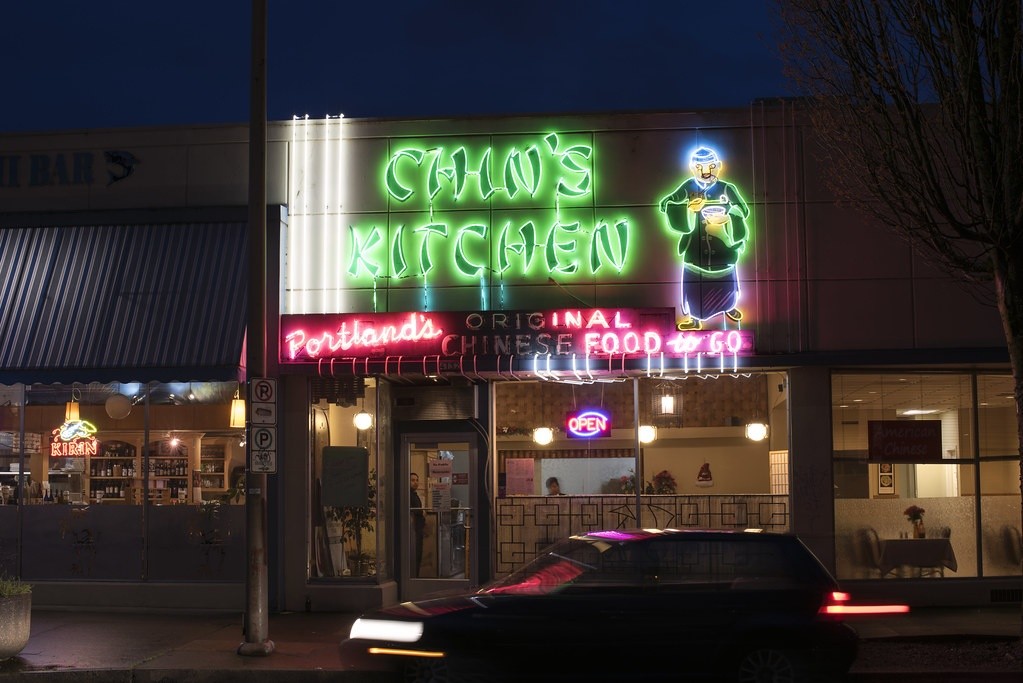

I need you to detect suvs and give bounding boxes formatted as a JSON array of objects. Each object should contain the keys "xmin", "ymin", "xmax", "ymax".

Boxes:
[{"xmin": 343, "ymin": 529, "xmax": 858, "ymax": 683}]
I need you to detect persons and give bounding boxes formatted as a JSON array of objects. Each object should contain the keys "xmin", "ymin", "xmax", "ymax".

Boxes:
[
  {"xmin": 546, "ymin": 477, "xmax": 565, "ymax": 495},
  {"xmin": 410, "ymin": 473, "xmax": 425, "ymax": 578}
]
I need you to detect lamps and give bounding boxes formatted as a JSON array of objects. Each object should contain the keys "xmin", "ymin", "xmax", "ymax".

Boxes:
[
  {"xmin": 353, "ymin": 385, "xmax": 373, "ymax": 430},
  {"xmin": 652, "ymin": 379, "xmax": 685, "ymax": 428},
  {"xmin": 105, "ymin": 381, "xmax": 131, "ymax": 419},
  {"xmin": 65, "ymin": 384, "xmax": 79, "ymax": 421},
  {"xmin": 229, "ymin": 382, "xmax": 246, "ymax": 428},
  {"xmin": 744, "ymin": 377, "xmax": 770, "ymax": 442}
]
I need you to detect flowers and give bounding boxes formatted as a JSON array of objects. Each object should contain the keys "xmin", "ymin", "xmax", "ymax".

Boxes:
[{"xmin": 904, "ymin": 505, "xmax": 925, "ymax": 522}]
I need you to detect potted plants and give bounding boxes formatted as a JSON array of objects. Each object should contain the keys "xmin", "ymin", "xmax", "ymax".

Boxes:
[
  {"xmin": 0, "ymin": 575, "xmax": 33, "ymax": 663},
  {"xmin": 227, "ymin": 476, "xmax": 246, "ymax": 504},
  {"xmin": 326, "ymin": 468, "xmax": 378, "ymax": 577}
]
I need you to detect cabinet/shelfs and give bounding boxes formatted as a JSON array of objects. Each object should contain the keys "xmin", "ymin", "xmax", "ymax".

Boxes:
[{"xmin": 41, "ymin": 430, "xmax": 235, "ymax": 504}]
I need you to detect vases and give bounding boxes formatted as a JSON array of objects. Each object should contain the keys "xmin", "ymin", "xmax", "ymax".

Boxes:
[{"xmin": 913, "ymin": 519, "xmax": 925, "ymax": 538}]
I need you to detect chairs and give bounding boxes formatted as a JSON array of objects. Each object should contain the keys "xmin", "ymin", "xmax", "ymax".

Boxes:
[
  {"xmin": 1005, "ymin": 526, "xmax": 1022, "ymax": 564},
  {"xmin": 862, "ymin": 526, "xmax": 951, "ymax": 578}
]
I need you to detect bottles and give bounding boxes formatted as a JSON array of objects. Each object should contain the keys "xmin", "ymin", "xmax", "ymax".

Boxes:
[
  {"xmin": 14, "ymin": 481, "xmax": 69, "ymax": 505},
  {"xmin": 90, "ymin": 443, "xmax": 188, "ymax": 498}
]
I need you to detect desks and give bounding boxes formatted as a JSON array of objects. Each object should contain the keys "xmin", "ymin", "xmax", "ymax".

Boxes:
[{"xmin": 879, "ymin": 539, "xmax": 957, "ymax": 578}]
